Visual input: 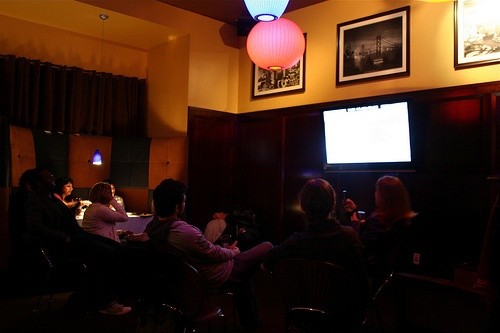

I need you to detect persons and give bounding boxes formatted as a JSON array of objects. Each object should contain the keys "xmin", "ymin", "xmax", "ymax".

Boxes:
[
  {"xmin": 102, "ymin": 179, "xmax": 125, "ymax": 212},
  {"xmin": 53, "ymin": 177, "xmax": 80, "ymax": 209},
  {"xmin": 9, "ymin": 169, "xmax": 38, "ymax": 282},
  {"xmin": 144, "ymin": 178, "xmax": 266, "ymax": 328},
  {"xmin": 281, "ymin": 177, "xmax": 369, "ymax": 333},
  {"xmin": 82, "ymin": 181, "xmax": 128, "ymax": 243},
  {"xmin": 364, "ymin": 175, "xmax": 423, "ymax": 255},
  {"xmin": 26, "ymin": 166, "xmax": 133, "ymax": 327}
]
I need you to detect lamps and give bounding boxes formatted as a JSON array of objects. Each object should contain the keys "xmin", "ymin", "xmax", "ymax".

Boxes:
[{"xmin": 92, "ymin": 149, "xmax": 102, "ymax": 166}]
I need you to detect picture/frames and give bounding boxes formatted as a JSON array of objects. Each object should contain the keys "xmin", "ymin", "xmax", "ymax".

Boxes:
[
  {"xmin": 336, "ymin": 5, "xmax": 411, "ymax": 86},
  {"xmin": 252, "ymin": 32, "xmax": 308, "ymax": 99},
  {"xmin": 454, "ymin": 0, "xmax": 500, "ymax": 69}
]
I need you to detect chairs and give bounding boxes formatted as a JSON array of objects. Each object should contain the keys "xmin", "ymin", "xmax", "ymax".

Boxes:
[{"xmin": 4, "ymin": 185, "xmax": 500, "ymax": 333}]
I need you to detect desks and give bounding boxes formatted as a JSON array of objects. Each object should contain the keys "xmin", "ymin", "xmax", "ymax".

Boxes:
[{"xmin": 75, "ymin": 200, "xmax": 155, "ymax": 235}]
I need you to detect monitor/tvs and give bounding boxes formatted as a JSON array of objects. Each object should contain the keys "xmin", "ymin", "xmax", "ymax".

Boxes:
[{"xmin": 320, "ymin": 97, "xmax": 416, "ymax": 170}]
